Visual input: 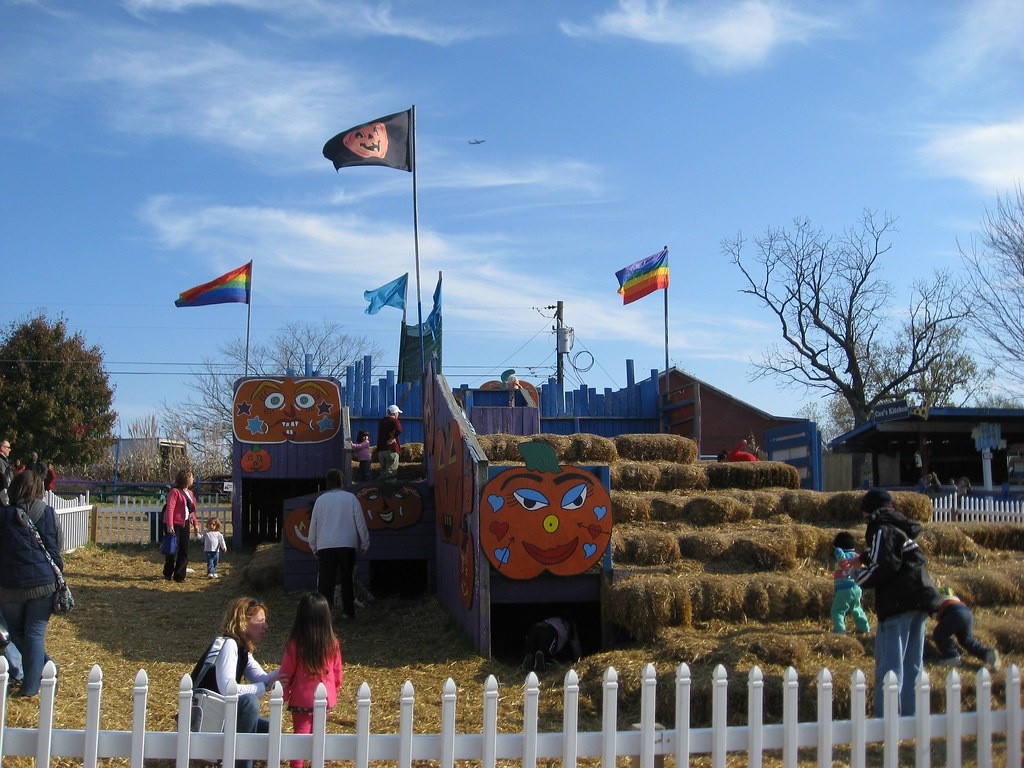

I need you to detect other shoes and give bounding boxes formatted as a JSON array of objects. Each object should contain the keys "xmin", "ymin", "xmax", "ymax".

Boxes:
[
  {"xmin": 208, "ymin": 573, "xmax": 219, "ymax": 579},
  {"xmin": 522, "ymin": 651, "xmax": 545, "ymax": 673},
  {"xmin": 165, "ymin": 575, "xmax": 171, "ymax": 580},
  {"xmin": 173, "ymin": 576, "xmax": 186, "ymax": 583},
  {"xmin": 985, "ymin": 648, "xmax": 1001, "ymax": 671},
  {"xmin": 939, "ymin": 656, "xmax": 962, "ymax": 667}
]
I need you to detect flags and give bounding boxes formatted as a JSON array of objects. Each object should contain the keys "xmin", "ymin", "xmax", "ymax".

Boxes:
[
  {"xmin": 615, "ymin": 249, "xmax": 670, "ymax": 306},
  {"xmin": 323, "ymin": 109, "xmax": 412, "ymax": 172},
  {"xmin": 175, "ymin": 261, "xmax": 251, "ymax": 308},
  {"xmin": 363, "ymin": 274, "xmax": 408, "ymax": 315}
]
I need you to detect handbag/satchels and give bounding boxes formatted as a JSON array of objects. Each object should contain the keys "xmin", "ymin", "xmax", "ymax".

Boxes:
[
  {"xmin": 54, "ymin": 579, "xmax": 75, "ymax": 616},
  {"xmin": 160, "ymin": 532, "xmax": 178, "ymax": 556},
  {"xmin": 162, "ymin": 504, "xmax": 167, "ymax": 512}
]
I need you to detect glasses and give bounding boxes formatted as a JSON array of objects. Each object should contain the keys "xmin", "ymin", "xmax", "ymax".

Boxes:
[
  {"xmin": 244, "ymin": 598, "xmax": 262, "ymax": 613},
  {"xmin": 4, "ymin": 446, "xmax": 11, "ymax": 449}
]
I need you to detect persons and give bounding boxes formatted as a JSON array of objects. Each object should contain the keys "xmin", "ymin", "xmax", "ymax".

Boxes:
[
  {"xmin": 929, "ymin": 586, "xmax": 1002, "ymax": 672},
  {"xmin": 521, "ymin": 608, "xmax": 579, "ymax": 673},
  {"xmin": 350, "ymin": 430, "xmax": 372, "ymax": 481},
  {"xmin": 308, "ymin": 468, "xmax": 369, "ymax": 618},
  {"xmin": 176, "ymin": 596, "xmax": 290, "ymax": 768},
  {"xmin": 830, "ymin": 486, "xmax": 936, "ymax": 719},
  {"xmin": 163, "ymin": 469, "xmax": 199, "ymax": 583},
  {"xmin": 924, "ymin": 471, "xmax": 971, "ymax": 521},
  {"xmin": 0, "ymin": 469, "xmax": 65, "ymax": 697},
  {"xmin": 0, "ymin": 438, "xmax": 57, "ymax": 507},
  {"xmin": 194, "ymin": 517, "xmax": 227, "ymax": 579},
  {"xmin": 376, "ymin": 404, "xmax": 403, "ymax": 480},
  {"xmin": 717, "ymin": 438, "xmax": 760, "ymax": 462},
  {"xmin": 279, "ymin": 592, "xmax": 342, "ymax": 768}
]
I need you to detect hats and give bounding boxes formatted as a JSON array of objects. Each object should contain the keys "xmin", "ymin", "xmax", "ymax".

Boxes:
[
  {"xmin": 860, "ymin": 487, "xmax": 891, "ymax": 508},
  {"xmin": 387, "ymin": 405, "xmax": 403, "ymax": 413},
  {"xmin": 832, "ymin": 531, "xmax": 856, "ymax": 551}
]
